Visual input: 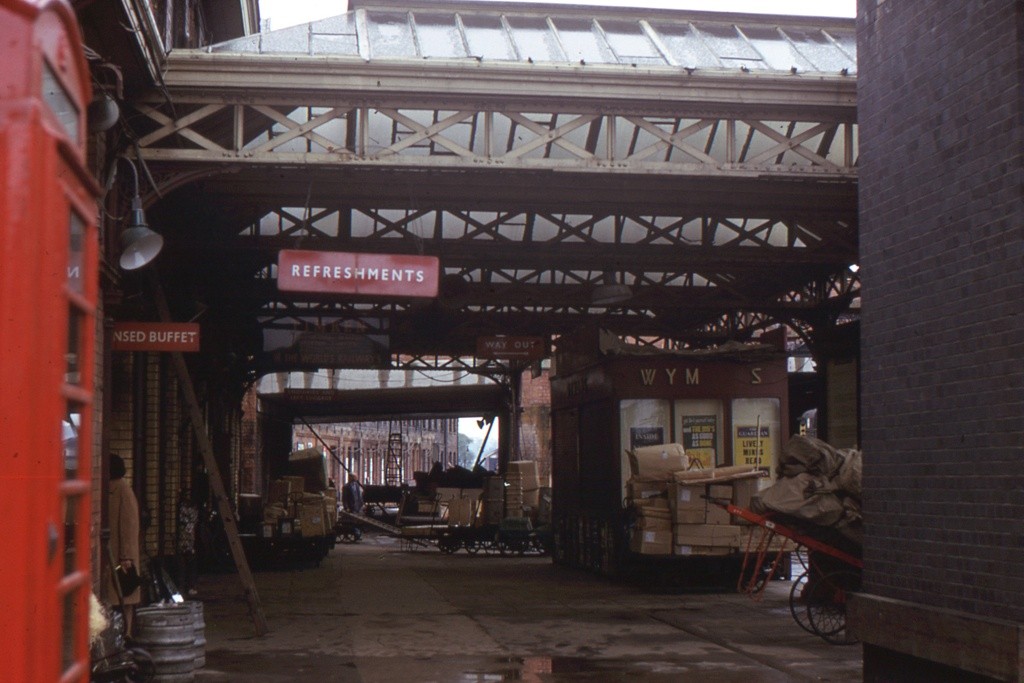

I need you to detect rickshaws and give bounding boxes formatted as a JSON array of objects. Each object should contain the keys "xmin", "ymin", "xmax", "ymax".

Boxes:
[
  {"xmin": 702, "ymin": 496, "xmax": 867, "ymax": 646},
  {"xmin": 435, "ymin": 525, "xmax": 548, "ymax": 557}
]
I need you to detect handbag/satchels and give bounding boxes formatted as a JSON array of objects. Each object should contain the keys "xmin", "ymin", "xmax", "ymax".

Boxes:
[{"xmin": 112, "ymin": 563, "xmax": 139, "ymax": 596}]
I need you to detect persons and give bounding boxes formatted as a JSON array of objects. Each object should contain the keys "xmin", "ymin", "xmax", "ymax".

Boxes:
[
  {"xmin": 107, "ymin": 454, "xmax": 141, "ymax": 637},
  {"xmin": 342, "ymin": 472, "xmax": 363, "ymax": 541}
]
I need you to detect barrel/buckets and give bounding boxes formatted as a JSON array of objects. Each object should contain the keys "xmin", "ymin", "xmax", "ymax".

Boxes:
[
  {"xmin": 134, "ymin": 604, "xmax": 196, "ymax": 683},
  {"xmin": 150, "ymin": 600, "xmax": 206, "ymax": 673}
]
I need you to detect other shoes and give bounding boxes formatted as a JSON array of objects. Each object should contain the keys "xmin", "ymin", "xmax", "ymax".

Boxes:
[{"xmin": 354, "ymin": 536, "xmax": 361, "ymax": 540}]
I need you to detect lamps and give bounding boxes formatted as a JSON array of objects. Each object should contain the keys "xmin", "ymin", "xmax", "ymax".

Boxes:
[
  {"xmin": 166, "ymin": 279, "xmax": 207, "ymax": 324},
  {"xmin": 99, "ymin": 153, "xmax": 163, "ymax": 270},
  {"xmin": 87, "ymin": 62, "xmax": 122, "ymax": 133}
]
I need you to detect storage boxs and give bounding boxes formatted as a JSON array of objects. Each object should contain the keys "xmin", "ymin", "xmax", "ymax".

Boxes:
[
  {"xmin": 624, "ymin": 442, "xmax": 808, "ymax": 556},
  {"xmin": 413, "ymin": 459, "xmax": 554, "ymax": 534},
  {"xmin": 233, "ymin": 447, "xmax": 338, "ymax": 537}
]
